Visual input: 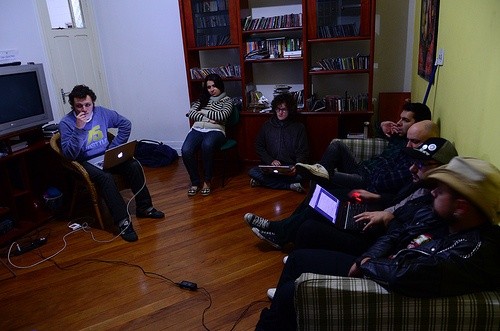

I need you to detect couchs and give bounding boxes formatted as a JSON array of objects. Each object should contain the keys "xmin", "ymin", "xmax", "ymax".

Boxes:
[{"xmin": 296, "ymin": 138, "xmax": 500, "ymax": 331}]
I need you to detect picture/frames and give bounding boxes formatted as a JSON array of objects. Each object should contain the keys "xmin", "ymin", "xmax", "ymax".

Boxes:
[{"xmin": 417, "ymin": 0, "xmax": 440, "ymax": 84}]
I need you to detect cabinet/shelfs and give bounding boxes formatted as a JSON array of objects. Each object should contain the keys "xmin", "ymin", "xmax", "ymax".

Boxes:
[
  {"xmin": 0, "ymin": 137, "xmax": 56, "ymax": 246},
  {"xmin": 178, "ymin": 0, "xmax": 375, "ymax": 172}
]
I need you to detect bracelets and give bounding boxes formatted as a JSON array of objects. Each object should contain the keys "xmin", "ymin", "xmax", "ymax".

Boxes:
[{"xmin": 356, "ymin": 255, "xmax": 371, "ymax": 270}]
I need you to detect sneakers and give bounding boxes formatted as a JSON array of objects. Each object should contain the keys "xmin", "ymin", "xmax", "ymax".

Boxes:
[
  {"xmin": 295, "ymin": 162, "xmax": 330, "ymax": 183},
  {"xmin": 251, "ymin": 227, "xmax": 283, "ymax": 251},
  {"xmin": 244, "ymin": 212, "xmax": 270, "ymax": 231}
]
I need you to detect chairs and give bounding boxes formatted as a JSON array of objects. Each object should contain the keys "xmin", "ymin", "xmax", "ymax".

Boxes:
[
  {"xmin": 50, "ymin": 130, "xmax": 135, "ymax": 231},
  {"xmin": 216, "ymin": 106, "xmax": 239, "ymax": 188}
]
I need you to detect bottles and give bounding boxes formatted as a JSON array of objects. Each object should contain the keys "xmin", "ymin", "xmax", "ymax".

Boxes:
[{"xmin": 332, "ymin": 93, "xmax": 368, "ymax": 112}]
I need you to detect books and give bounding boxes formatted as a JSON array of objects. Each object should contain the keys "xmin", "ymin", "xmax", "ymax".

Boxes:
[{"xmin": 190, "ymin": 0, "xmax": 368, "ymax": 112}]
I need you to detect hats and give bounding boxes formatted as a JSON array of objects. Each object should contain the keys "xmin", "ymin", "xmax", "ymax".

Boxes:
[
  {"xmin": 425, "ymin": 156, "xmax": 500, "ymax": 228},
  {"xmin": 410, "ymin": 137, "xmax": 459, "ymax": 166}
]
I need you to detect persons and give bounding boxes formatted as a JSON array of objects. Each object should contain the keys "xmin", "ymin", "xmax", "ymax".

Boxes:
[
  {"xmin": 242, "ymin": 103, "xmax": 500, "ymax": 331},
  {"xmin": 57, "ymin": 85, "xmax": 163, "ymax": 242},
  {"xmin": 180, "ymin": 75, "xmax": 234, "ymax": 197},
  {"xmin": 248, "ymin": 94, "xmax": 310, "ymax": 194}
]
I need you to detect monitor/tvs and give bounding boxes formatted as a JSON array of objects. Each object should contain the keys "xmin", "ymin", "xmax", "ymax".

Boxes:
[{"xmin": 0, "ymin": 63, "xmax": 54, "ymax": 152}]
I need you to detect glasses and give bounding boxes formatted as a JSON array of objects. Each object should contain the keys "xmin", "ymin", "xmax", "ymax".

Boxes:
[{"xmin": 275, "ymin": 107, "xmax": 288, "ymax": 113}]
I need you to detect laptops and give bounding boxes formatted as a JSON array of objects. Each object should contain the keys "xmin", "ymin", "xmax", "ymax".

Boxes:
[
  {"xmin": 86, "ymin": 140, "xmax": 137, "ymax": 170},
  {"xmin": 306, "ymin": 182, "xmax": 373, "ymax": 233},
  {"xmin": 16, "ymin": 236, "xmax": 47, "ymax": 254},
  {"xmin": 258, "ymin": 164, "xmax": 292, "ymax": 174}
]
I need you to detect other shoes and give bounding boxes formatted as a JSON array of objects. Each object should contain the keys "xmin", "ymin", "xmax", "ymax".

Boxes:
[
  {"xmin": 266, "ymin": 287, "xmax": 277, "ymax": 300},
  {"xmin": 119, "ymin": 219, "xmax": 138, "ymax": 242},
  {"xmin": 249, "ymin": 178, "xmax": 257, "ymax": 187},
  {"xmin": 135, "ymin": 207, "xmax": 164, "ymax": 219},
  {"xmin": 289, "ymin": 182, "xmax": 304, "ymax": 193},
  {"xmin": 200, "ymin": 187, "xmax": 212, "ymax": 196},
  {"xmin": 188, "ymin": 186, "xmax": 200, "ymax": 196}
]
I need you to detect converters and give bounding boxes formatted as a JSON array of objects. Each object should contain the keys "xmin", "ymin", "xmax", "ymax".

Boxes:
[{"xmin": 179, "ymin": 281, "xmax": 197, "ymax": 291}]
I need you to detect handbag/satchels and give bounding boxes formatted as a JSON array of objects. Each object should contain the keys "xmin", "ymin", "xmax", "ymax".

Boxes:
[{"xmin": 134, "ymin": 139, "xmax": 178, "ymax": 168}]
